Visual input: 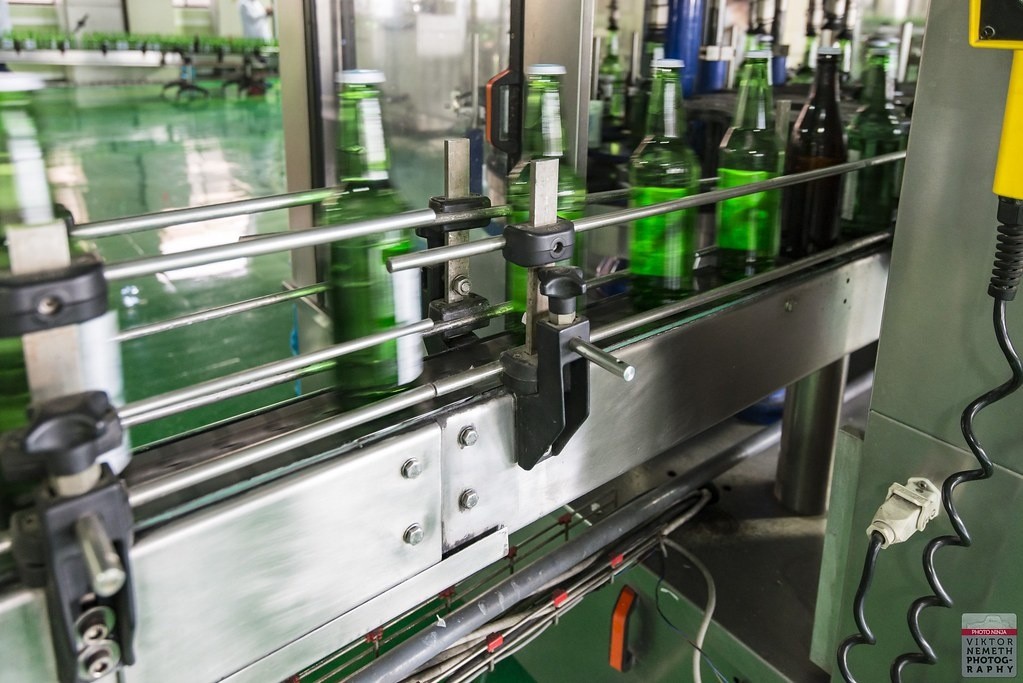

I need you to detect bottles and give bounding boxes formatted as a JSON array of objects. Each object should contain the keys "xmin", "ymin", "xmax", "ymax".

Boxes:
[
  {"xmin": 598, "ymin": 22, "xmax": 922, "ymax": 306},
  {"xmin": 0, "ymin": 72, "xmax": 132, "ymax": 515},
  {"xmin": 505, "ymin": 64, "xmax": 587, "ymax": 339},
  {"xmin": 325, "ymin": 67, "xmax": 425, "ymax": 403}
]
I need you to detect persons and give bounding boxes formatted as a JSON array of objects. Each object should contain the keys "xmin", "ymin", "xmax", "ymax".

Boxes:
[
  {"xmin": 160, "ymin": 57, "xmax": 196, "ymax": 98},
  {"xmin": 240, "ymin": 47, "xmax": 274, "ymax": 90}
]
